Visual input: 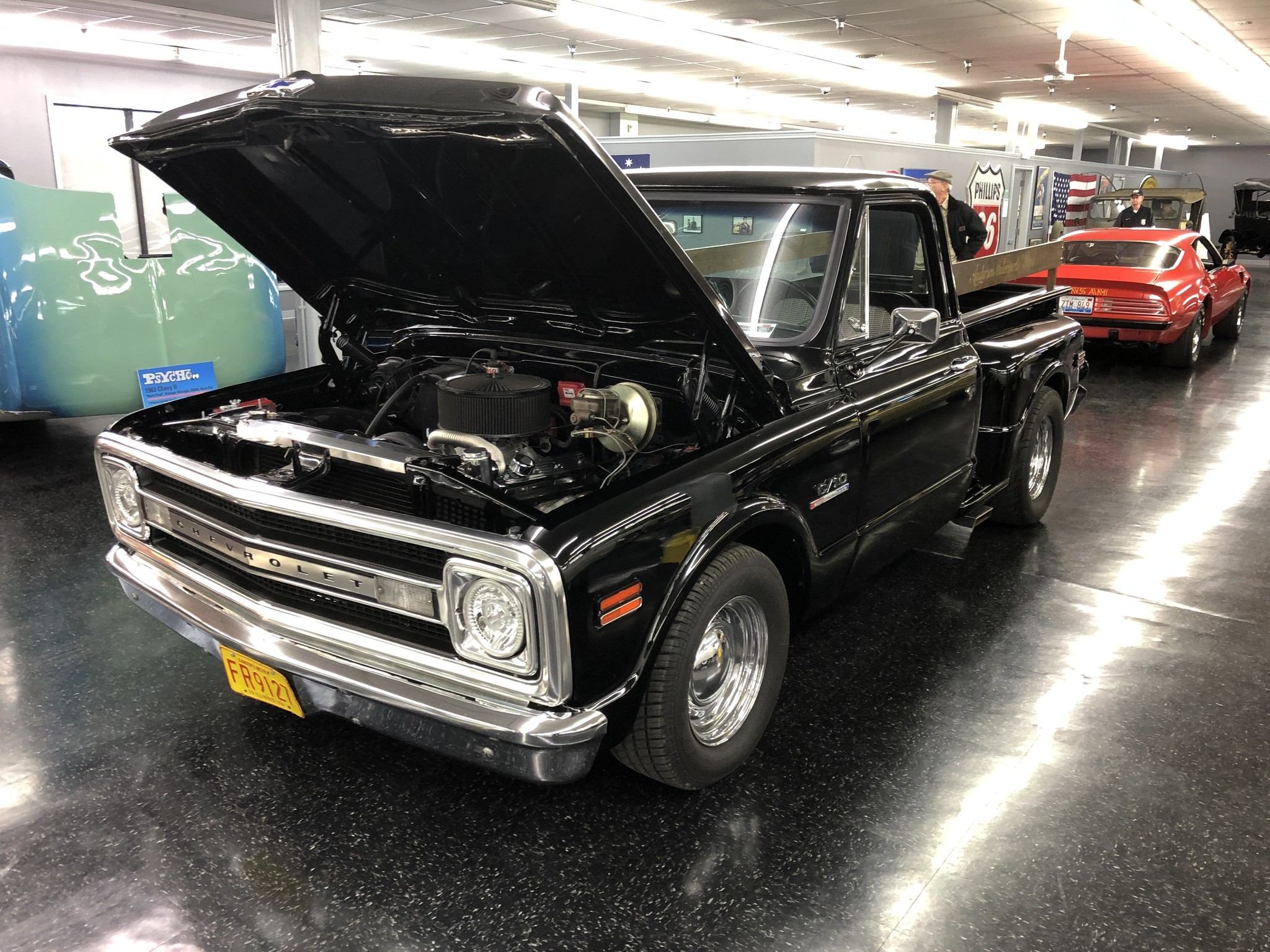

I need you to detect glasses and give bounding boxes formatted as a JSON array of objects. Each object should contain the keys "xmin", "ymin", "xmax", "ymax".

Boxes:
[
  {"xmin": 1131, "ymin": 196, "xmax": 1142, "ymax": 199},
  {"xmin": 926, "ymin": 180, "xmax": 944, "ymax": 185}
]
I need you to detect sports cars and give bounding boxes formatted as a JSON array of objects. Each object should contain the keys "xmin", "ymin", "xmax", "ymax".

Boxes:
[{"xmin": 1003, "ymin": 227, "xmax": 1251, "ymax": 373}]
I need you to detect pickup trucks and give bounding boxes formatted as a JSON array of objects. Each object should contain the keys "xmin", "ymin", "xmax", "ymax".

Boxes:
[{"xmin": 93, "ymin": 71, "xmax": 1089, "ymax": 796}]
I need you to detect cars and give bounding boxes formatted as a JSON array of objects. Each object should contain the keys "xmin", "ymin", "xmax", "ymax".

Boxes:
[{"xmin": 1085, "ymin": 172, "xmax": 1207, "ymax": 255}]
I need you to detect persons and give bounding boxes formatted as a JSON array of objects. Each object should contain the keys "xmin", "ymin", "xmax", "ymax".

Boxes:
[
  {"xmin": 1037, "ymin": 182, "xmax": 1043, "ymax": 205},
  {"xmin": 922, "ymin": 171, "xmax": 987, "ymax": 263},
  {"xmin": 1113, "ymin": 189, "xmax": 1156, "ymax": 228},
  {"xmin": 1153, "ymin": 200, "xmax": 1178, "ymax": 217}
]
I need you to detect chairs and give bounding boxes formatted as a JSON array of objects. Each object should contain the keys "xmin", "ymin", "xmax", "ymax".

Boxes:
[
  {"xmin": 1142, "ymin": 251, "xmax": 1178, "ymax": 271},
  {"xmin": 1088, "ymin": 252, "xmax": 1121, "ymax": 265},
  {"xmin": 768, "ymin": 280, "xmax": 922, "ymax": 339}
]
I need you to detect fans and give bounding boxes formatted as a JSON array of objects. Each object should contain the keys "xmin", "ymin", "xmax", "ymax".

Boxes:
[{"xmin": 978, "ymin": 20, "xmax": 1153, "ymax": 89}]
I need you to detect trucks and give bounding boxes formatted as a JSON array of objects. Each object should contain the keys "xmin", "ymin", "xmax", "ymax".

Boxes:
[{"xmin": 1217, "ymin": 178, "xmax": 1270, "ymax": 262}]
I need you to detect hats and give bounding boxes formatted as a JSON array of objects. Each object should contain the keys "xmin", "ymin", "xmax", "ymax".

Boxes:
[
  {"xmin": 924, "ymin": 170, "xmax": 954, "ymax": 185},
  {"xmin": 1129, "ymin": 189, "xmax": 1143, "ymax": 198}
]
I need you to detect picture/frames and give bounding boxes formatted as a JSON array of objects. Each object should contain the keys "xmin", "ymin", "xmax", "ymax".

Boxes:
[
  {"xmin": 682, "ymin": 214, "xmax": 703, "ymax": 234},
  {"xmin": 1029, "ymin": 238, "xmax": 1043, "ymax": 247},
  {"xmin": 732, "ymin": 216, "xmax": 753, "ymax": 235}
]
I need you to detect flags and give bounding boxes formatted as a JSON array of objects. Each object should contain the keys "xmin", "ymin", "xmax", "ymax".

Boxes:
[{"xmin": 1048, "ymin": 171, "xmax": 1097, "ymax": 242}]
